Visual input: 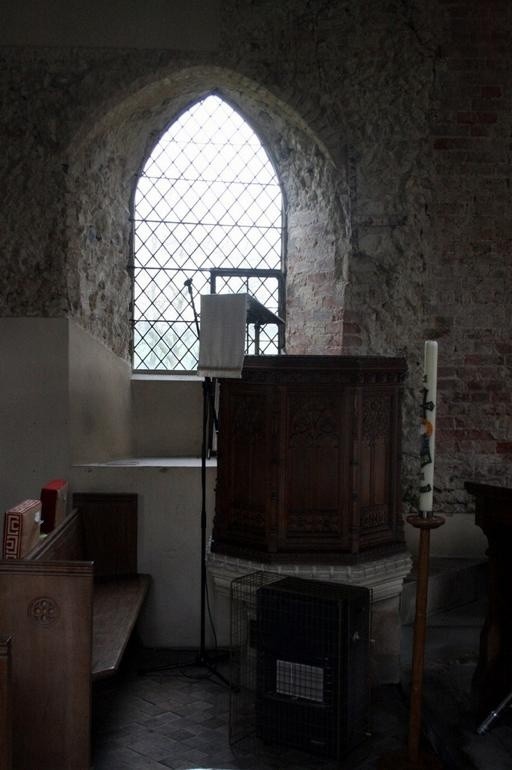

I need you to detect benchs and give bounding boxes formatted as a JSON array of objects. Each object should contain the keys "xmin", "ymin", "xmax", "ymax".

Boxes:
[{"xmin": 0, "ymin": 489, "xmax": 157, "ymax": 770}]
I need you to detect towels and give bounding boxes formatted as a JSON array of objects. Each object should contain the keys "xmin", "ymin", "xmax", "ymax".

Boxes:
[{"xmin": 196, "ymin": 292, "xmax": 250, "ymax": 378}]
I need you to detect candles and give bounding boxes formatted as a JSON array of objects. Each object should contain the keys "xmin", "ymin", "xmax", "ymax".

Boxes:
[{"xmin": 419, "ymin": 336, "xmax": 438, "ymax": 515}]
